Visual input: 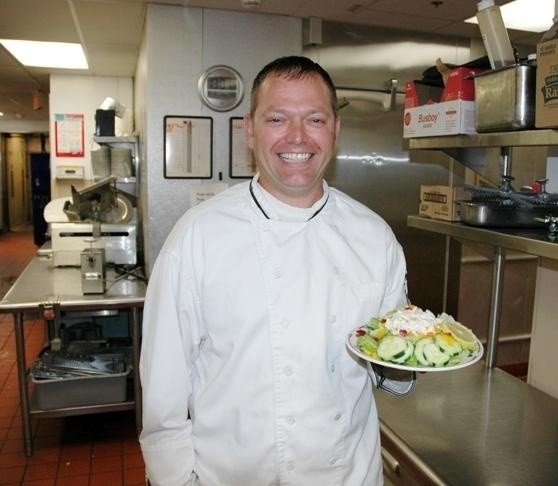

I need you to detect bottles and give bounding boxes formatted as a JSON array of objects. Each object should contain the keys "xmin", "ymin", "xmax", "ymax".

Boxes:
[
  {"xmin": 404, "ymin": 80, "xmax": 420, "ymax": 109},
  {"xmin": 475, "ymin": 0, "xmax": 516, "ymax": 70}
]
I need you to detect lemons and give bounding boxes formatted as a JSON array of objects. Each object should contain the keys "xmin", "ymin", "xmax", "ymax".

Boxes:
[{"xmin": 442, "ymin": 319, "xmax": 477, "ymax": 347}]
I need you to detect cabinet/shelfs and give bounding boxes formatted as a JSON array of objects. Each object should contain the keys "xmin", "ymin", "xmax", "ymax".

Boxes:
[{"xmin": 408, "ymin": 130, "xmax": 558, "ymax": 261}]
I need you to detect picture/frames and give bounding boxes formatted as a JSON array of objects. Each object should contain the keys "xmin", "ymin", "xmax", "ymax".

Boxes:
[{"xmin": 163, "ymin": 115, "xmax": 257, "ymax": 179}]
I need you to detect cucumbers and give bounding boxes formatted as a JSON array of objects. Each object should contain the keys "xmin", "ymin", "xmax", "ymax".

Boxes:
[{"xmin": 378, "ymin": 334, "xmax": 461, "ymax": 369}]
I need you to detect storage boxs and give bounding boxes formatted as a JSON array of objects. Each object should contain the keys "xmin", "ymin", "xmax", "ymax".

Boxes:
[{"xmin": 535, "ymin": 25, "xmax": 558, "ymax": 128}]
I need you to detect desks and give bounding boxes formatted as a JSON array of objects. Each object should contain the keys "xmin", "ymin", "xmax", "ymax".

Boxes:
[{"xmin": 1, "ymin": 257, "xmax": 147, "ymax": 457}]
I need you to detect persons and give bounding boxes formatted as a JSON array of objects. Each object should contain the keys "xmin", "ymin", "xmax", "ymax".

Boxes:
[{"xmin": 137, "ymin": 56, "xmax": 428, "ymax": 486}]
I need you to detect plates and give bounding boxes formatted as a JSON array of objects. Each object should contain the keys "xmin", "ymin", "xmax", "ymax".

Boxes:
[{"xmin": 346, "ymin": 316, "xmax": 485, "ymax": 373}]
